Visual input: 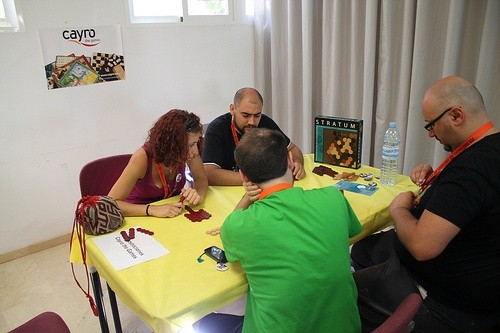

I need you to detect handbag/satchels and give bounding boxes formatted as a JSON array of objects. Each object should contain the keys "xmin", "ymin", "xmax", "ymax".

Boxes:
[{"xmin": 77, "ymin": 195, "xmax": 124, "ymax": 235}]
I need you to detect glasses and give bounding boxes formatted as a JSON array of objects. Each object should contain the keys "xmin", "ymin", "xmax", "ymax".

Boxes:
[{"xmin": 424, "ymin": 106, "xmax": 462, "ymax": 131}]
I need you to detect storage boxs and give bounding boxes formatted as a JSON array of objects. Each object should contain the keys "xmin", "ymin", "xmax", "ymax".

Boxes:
[{"xmin": 314, "ymin": 115, "xmax": 363, "ymax": 169}]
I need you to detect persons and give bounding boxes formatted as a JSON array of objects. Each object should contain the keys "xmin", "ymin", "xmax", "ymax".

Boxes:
[
  {"xmin": 105, "ymin": 109, "xmax": 208, "ymax": 217},
  {"xmin": 200, "ymin": 87, "xmax": 307, "ymax": 186},
  {"xmin": 352, "ymin": 77, "xmax": 500, "ymax": 333},
  {"xmin": 191, "ymin": 127, "xmax": 364, "ymax": 333}
]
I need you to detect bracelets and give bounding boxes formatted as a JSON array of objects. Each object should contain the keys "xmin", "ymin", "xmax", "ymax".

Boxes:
[{"xmin": 146, "ymin": 205, "xmax": 151, "ymax": 216}]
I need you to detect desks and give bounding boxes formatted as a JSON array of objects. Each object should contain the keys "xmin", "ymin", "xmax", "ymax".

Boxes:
[{"xmin": 69, "ymin": 153, "xmax": 420, "ymax": 333}]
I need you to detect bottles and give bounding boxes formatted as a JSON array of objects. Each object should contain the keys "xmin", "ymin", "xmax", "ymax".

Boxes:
[{"xmin": 380, "ymin": 122, "xmax": 401, "ymax": 186}]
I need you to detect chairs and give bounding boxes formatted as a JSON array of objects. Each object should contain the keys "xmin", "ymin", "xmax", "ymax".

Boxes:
[{"xmin": 79, "ymin": 154, "xmax": 132, "ymax": 333}]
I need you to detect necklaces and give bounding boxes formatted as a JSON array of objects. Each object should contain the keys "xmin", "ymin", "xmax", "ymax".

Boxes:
[{"xmin": 166, "ymin": 164, "xmax": 176, "ymax": 175}]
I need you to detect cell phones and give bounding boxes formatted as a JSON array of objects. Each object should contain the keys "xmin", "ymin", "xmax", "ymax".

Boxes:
[{"xmin": 203, "ymin": 245, "xmax": 227, "ymax": 263}]
